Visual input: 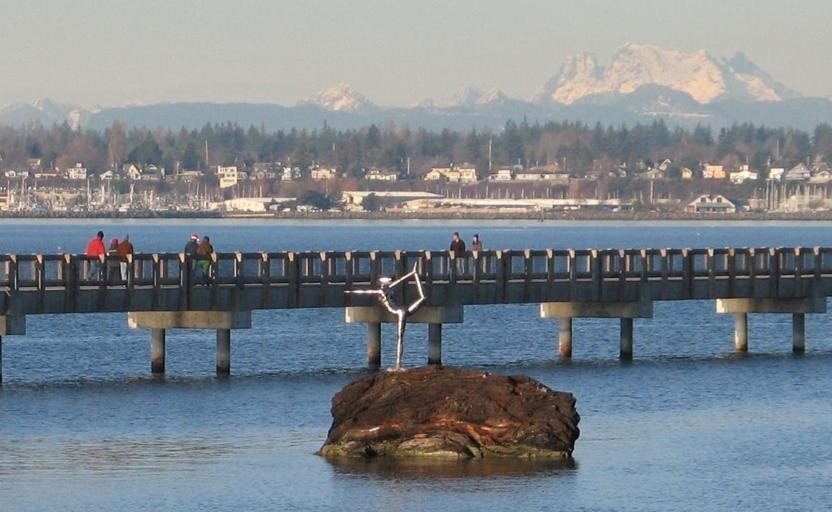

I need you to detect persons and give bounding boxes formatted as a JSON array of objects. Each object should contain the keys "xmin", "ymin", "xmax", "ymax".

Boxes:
[
  {"xmin": 117, "ymin": 233, "xmax": 134, "ymax": 283},
  {"xmin": 107, "ymin": 238, "xmax": 120, "ymax": 283},
  {"xmin": 196, "ymin": 236, "xmax": 216, "ymax": 284},
  {"xmin": 471, "ymin": 233, "xmax": 483, "ymax": 274},
  {"xmin": 449, "ymin": 232, "xmax": 466, "ymax": 280},
  {"xmin": 86, "ymin": 230, "xmax": 107, "ymax": 282},
  {"xmin": 185, "ymin": 232, "xmax": 200, "ymax": 284}
]
